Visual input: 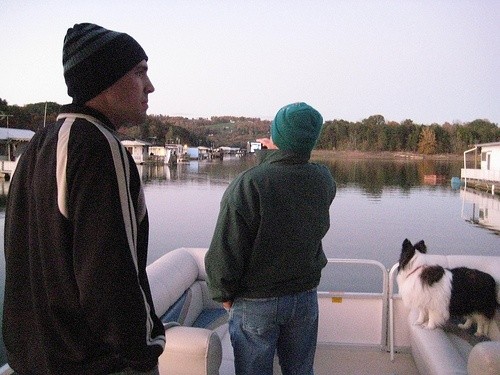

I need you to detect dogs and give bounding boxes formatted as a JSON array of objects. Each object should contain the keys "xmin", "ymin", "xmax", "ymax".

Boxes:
[{"xmin": 396, "ymin": 238, "xmax": 500, "ymax": 337}]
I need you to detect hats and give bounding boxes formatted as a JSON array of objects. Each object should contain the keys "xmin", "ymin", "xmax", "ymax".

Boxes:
[
  {"xmin": 62, "ymin": 22, "xmax": 148, "ymax": 104},
  {"xmin": 271, "ymin": 102, "xmax": 323, "ymax": 153}
]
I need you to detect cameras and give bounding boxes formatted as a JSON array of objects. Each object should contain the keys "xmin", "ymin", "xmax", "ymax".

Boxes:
[{"xmin": 246, "ymin": 141, "xmax": 268, "ymax": 153}]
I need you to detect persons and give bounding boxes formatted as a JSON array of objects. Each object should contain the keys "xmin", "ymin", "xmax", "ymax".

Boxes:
[
  {"xmin": 1, "ymin": 22, "xmax": 166, "ymax": 375},
  {"xmin": 203, "ymin": 102, "xmax": 336, "ymax": 375}
]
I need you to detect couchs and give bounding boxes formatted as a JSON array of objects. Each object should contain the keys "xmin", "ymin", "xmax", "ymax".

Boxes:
[
  {"xmin": 146, "ymin": 248, "xmax": 238, "ymax": 375},
  {"xmin": 409, "ymin": 255, "xmax": 500, "ymax": 375}
]
